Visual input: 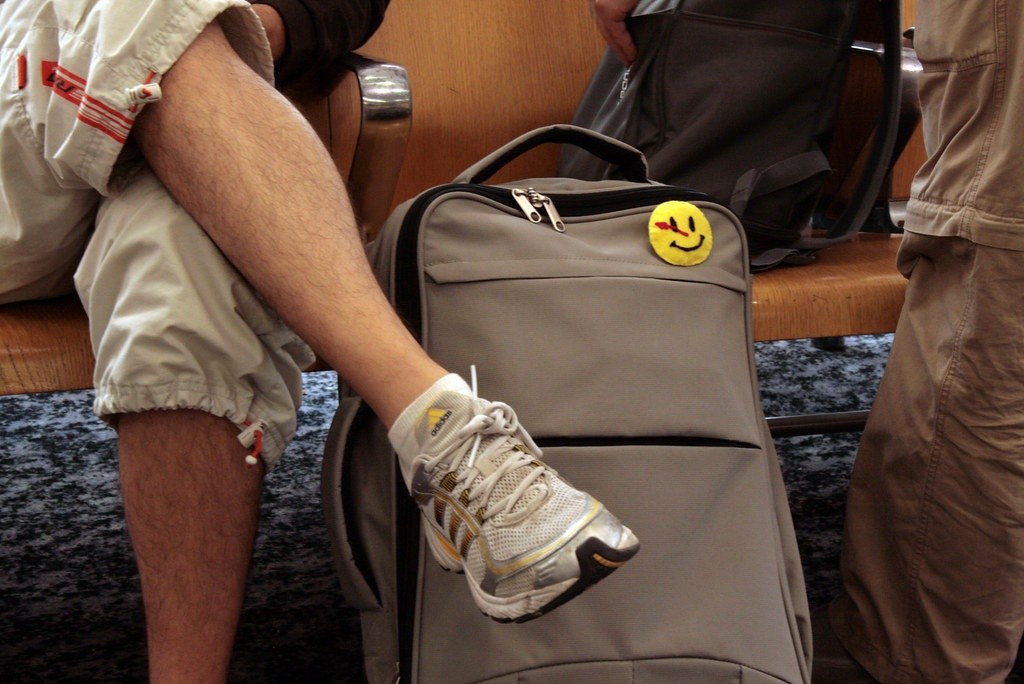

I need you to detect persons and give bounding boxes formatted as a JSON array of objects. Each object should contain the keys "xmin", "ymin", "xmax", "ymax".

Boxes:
[
  {"xmin": 589, "ymin": 0, "xmax": 1024, "ymax": 684},
  {"xmin": 0, "ymin": 0, "xmax": 640, "ymax": 684}
]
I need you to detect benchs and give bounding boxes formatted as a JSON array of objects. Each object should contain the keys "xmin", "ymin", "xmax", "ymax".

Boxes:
[{"xmin": 0, "ymin": 0, "xmax": 925, "ymax": 395}]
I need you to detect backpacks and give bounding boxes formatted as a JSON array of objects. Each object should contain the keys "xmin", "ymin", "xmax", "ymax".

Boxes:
[
  {"xmin": 320, "ymin": 124, "xmax": 813, "ymax": 684},
  {"xmin": 559, "ymin": 0, "xmax": 902, "ymax": 271}
]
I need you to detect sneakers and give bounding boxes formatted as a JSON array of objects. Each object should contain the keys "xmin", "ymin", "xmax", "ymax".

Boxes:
[{"xmin": 410, "ymin": 366, "xmax": 640, "ymax": 625}]
